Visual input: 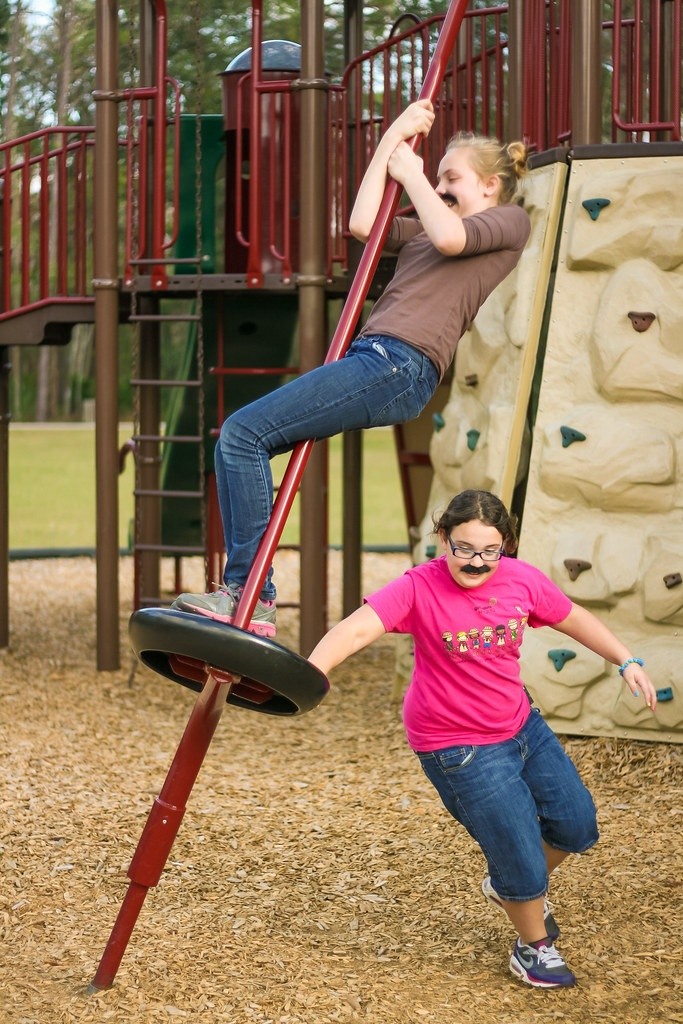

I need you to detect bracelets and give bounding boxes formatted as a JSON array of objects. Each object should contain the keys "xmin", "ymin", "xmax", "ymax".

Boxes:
[{"xmin": 618, "ymin": 657, "xmax": 643, "ymax": 676}]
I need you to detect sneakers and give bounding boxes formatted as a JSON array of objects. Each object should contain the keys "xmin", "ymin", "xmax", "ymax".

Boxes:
[
  {"xmin": 481, "ymin": 877, "xmax": 561, "ymax": 941},
  {"xmin": 170, "ymin": 581, "xmax": 278, "ymax": 638},
  {"xmin": 509, "ymin": 936, "xmax": 576, "ymax": 988}
]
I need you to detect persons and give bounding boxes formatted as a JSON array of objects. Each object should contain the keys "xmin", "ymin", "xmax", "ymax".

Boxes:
[
  {"xmin": 168, "ymin": 98, "xmax": 532, "ymax": 638},
  {"xmin": 305, "ymin": 490, "xmax": 656, "ymax": 989}
]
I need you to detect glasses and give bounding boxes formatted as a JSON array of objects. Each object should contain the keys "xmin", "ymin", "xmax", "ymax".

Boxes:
[{"xmin": 447, "ymin": 533, "xmax": 507, "ymax": 561}]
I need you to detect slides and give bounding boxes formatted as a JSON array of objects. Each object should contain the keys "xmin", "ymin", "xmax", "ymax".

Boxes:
[{"xmin": 127, "ymin": 294, "xmax": 301, "ymax": 559}]
